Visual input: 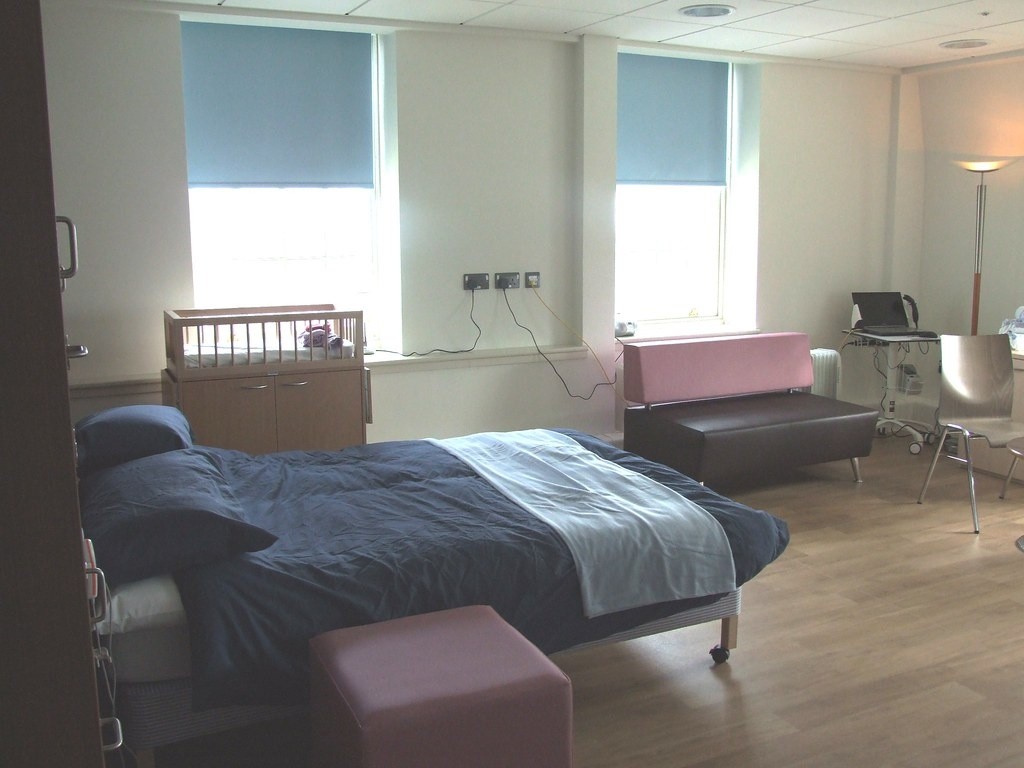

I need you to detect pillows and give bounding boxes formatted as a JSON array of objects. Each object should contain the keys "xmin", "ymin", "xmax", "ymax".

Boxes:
[
  {"xmin": 73, "ymin": 403, "xmax": 193, "ymax": 467},
  {"xmin": 76, "ymin": 447, "xmax": 279, "ymax": 591}
]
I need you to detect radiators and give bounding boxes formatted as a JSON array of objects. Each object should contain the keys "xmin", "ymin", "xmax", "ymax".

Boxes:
[{"xmin": 792, "ymin": 347, "xmax": 840, "ymax": 400}]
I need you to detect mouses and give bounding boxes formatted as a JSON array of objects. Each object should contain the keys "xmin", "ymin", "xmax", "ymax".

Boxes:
[{"xmin": 920, "ymin": 330, "xmax": 936, "ymax": 338}]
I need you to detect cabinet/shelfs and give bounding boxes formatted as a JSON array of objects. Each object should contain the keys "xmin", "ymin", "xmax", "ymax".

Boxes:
[{"xmin": 160, "ymin": 304, "xmax": 372, "ymax": 456}]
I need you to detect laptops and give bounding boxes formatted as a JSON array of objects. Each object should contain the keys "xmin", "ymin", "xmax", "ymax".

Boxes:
[{"xmin": 852, "ymin": 292, "xmax": 930, "ymax": 335}]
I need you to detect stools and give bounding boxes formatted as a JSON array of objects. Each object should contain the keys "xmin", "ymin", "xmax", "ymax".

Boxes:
[
  {"xmin": 1008, "ymin": 437, "xmax": 1024, "ymax": 551},
  {"xmin": 306, "ymin": 603, "xmax": 575, "ymax": 768}
]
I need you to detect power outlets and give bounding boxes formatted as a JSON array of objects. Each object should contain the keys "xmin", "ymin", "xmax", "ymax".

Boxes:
[
  {"xmin": 495, "ymin": 272, "xmax": 520, "ymax": 289},
  {"xmin": 463, "ymin": 273, "xmax": 490, "ymax": 290},
  {"xmin": 525, "ymin": 272, "xmax": 541, "ymax": 288}
]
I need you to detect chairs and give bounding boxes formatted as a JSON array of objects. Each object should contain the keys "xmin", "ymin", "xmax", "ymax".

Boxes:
[{"xmin": 916, "ymin": 334, "xmax": 1024, "ymax": 533}]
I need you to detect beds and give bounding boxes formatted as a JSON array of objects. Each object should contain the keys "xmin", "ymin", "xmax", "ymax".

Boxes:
[{"xmin": 90, "ymin": 428, "xmax": 790, "ymax": 767}]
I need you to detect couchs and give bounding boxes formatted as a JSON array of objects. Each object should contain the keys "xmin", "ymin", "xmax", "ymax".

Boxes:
[{"xmin": 623, "ymin": 331, "xmax": 880, "ymax": 495}]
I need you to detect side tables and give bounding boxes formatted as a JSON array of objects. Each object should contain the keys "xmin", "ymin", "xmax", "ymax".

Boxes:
[{"xmin": 840, "ymin": 329, "xmax": 943, "ymax": 454}]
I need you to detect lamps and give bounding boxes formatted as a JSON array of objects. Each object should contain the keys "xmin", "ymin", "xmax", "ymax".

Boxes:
[{"xmin": 952, "ymin": 155, "xmax": 1011, "ymax": 335}]
